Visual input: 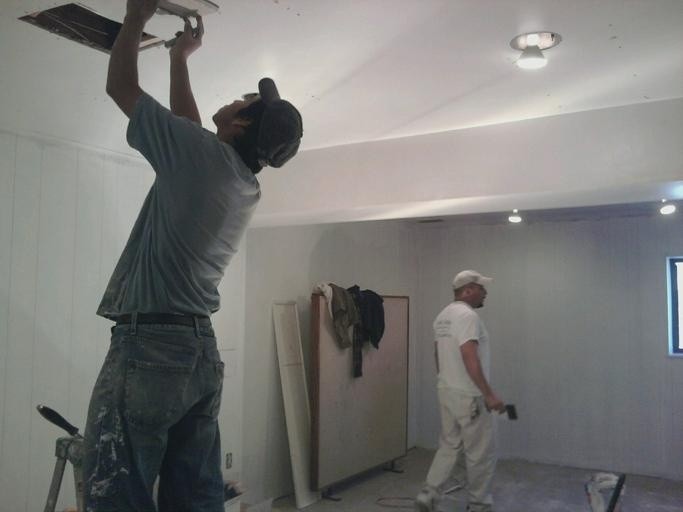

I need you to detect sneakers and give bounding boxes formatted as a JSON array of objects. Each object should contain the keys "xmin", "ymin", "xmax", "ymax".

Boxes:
[{"xmin": 416, "ymin": 493, "xmax": 441, "ymax": 512}]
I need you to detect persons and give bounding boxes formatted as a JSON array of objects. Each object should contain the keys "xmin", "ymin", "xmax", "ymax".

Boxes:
[
  {"xmin": 413, "ymin": 269, "xmax": 507, "ymax": 512},
  {"xmin": 80, "ymin": 1, "xmax": 306, "ymax": 512}
]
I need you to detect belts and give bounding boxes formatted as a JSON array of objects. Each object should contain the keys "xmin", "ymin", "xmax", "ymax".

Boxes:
[{"xmin": 116, "ymin": 314, "xmax": 213, "ymax": 329}]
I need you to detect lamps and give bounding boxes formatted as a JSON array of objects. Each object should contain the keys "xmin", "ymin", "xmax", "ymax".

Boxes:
[
  {"xmin": 660, "ymin": 201, "xmax": 676, "ymax": 215},
  {"xmin": 515, "ymin": 44, "xmax": 548, "ymax": 69},
  {"xmin": 508, "ymin": 212, "xmax": 522, "ymax": 223}
]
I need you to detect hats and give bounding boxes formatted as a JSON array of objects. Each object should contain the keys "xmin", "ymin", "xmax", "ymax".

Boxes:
[
  {"xmin": 453, "ymin": 270, "xmax": 492, "ymax": 291},
  {"xmin": 255, "ymin": 78, "xmax": 303, "ymax": 170}
]
